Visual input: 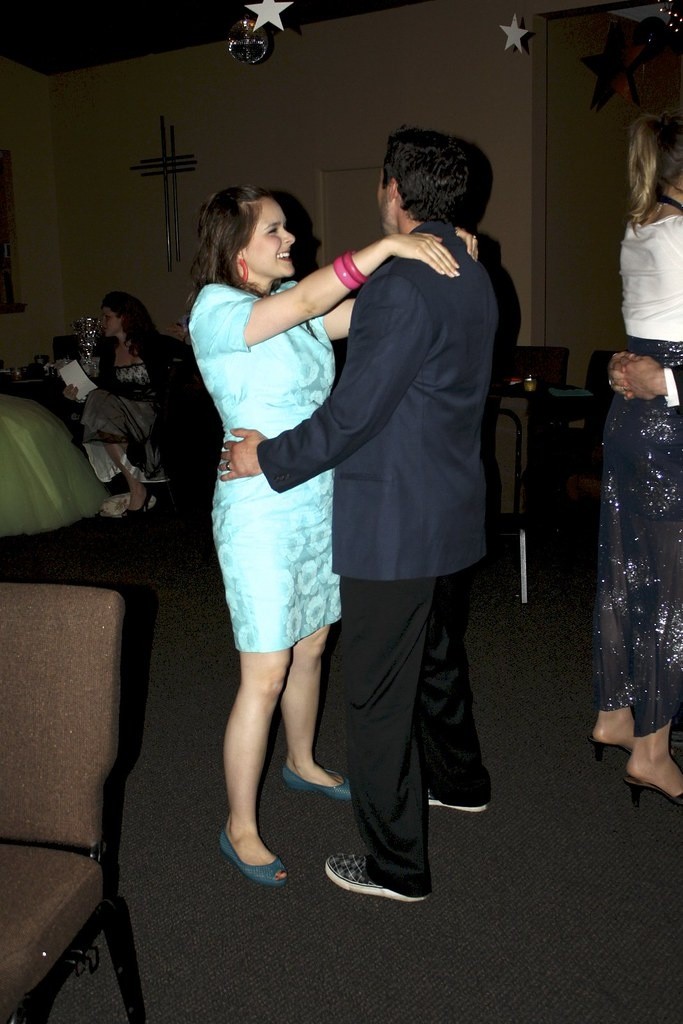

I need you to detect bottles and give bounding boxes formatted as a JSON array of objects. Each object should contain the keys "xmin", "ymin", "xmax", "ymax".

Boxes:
[{"xmin": 49, "ymin": 365, "xmax": 55, "ymax": 375}]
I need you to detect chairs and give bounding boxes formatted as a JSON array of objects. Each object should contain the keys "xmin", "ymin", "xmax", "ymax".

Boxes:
[
  {"xmin": 515, "ymin": 345, "xmax": 569, "ymax": 384},
  {"xmin": 585, "ymin": 350, "xmax": 626, "ymax": 391},
  {"xmin": 0, "ymin": 582, "xmax": 160, "ymax": 1024},
  {"xmin": 480, "ymin": 395, "xmax": 529, "ymax": 606}
]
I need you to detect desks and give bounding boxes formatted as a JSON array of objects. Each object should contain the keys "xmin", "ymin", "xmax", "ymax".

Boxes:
[{"xmin": 479, "ymin": 382, "xmax": 594, "ymax": 425}]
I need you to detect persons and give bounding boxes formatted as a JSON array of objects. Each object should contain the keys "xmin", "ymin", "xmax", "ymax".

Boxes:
[
  {"xmin": 217, "ymin": 121, "xmax": 500, "ymax": 903},
  {"xmin": 587, "ymin": 107, "xmax": 683, "ymax": 809},
  {"xmin": 62, "ymin": 290, "xmax": 173, "ymax": 523},
  {"xmin": 188, "ymin": 183, "xmax": 477, "ymax": 881}
]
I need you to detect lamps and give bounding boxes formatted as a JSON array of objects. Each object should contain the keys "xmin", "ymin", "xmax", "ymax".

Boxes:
[{"xmin": 228, "ymin": 14, "xmax": 269, "ymax": 64}]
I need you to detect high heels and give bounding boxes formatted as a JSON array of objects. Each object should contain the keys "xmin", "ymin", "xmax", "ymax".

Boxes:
[
  {"xmin": 623, "ymin": 774, "xmax": 683, "ymax": 807},
  {"xmin": 121, "ymin": 488, "xmax": 151, "ymax": 522},
  {"xmin": 588, "ymin": 734, "xmax": 632, "ymax": 762}
]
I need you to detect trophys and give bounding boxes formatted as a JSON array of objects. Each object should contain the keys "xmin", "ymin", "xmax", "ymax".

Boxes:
[{"xmin": 71, "ymin": 314, "xmax": 102, "ymax": 376}]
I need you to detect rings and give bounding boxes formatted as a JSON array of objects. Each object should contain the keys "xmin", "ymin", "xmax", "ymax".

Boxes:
[
  {"xmin": 621, "ymin": 387, "xmax": 629, "ymax": 391},
  {"xmin": 609, "ymin": 379, "xmax": 612, "ymax": 385},
  {"xmin": 226, "ymin": 462, "xmax": 231, "ymax": 470},
  {"xmin": 473, "ymin": 235, "xmax": 476, "ymax": 238}
]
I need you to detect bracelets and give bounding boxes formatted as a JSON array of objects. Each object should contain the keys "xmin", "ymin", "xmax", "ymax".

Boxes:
[{"xmin": 333, "ymin": 249, "xmax": 368, "ymax": 290}]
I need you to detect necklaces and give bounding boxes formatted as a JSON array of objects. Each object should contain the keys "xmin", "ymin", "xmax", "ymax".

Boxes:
[{"xmin": 656, "ymin": 203, "xmax": 669, "ymax": 213}]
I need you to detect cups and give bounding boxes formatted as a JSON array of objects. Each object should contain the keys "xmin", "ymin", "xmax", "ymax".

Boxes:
[{"xmin": 523, "ymin": 373, "xmax": 536, "ymax": 391}]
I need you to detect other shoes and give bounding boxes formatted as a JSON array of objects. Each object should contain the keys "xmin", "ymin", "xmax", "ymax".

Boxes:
[
  {"xmin": 325, "ymin": 853, "xmax": 429, "ymax": 902},
  {"xmin": 426, "ymin": 785, "xmax": 487, "ymax": 812}
]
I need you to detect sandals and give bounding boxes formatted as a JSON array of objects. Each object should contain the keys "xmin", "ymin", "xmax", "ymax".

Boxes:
[
  {"xmin": 283, "ymin": 764, "xmax": 352, "ymax": 800},
  {"xmin": 219, "ymin": 827, "xmax": 287, "ymax": 887}
]
